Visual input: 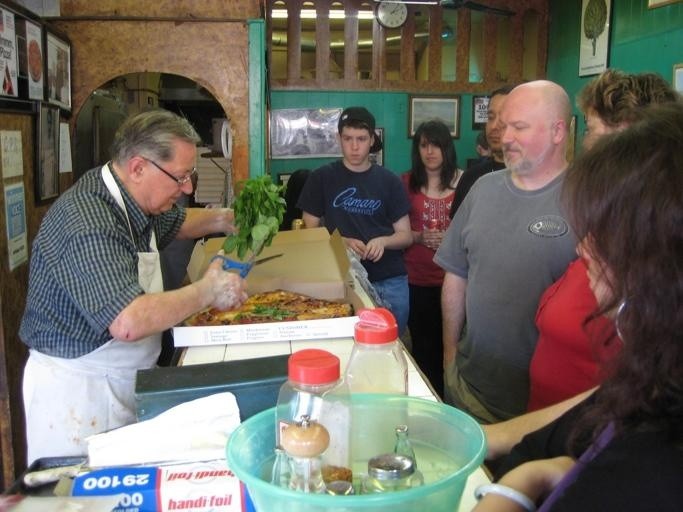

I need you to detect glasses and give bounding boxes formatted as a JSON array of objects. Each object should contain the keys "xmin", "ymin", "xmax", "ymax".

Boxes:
[{"xmin": 136, "ymin": 156, "xmax": 197, "ymax": 185}]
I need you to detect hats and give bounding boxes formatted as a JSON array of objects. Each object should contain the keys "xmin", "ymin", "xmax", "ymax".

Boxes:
[{"xmin": 339, "ymin": 106, "xmax": 384, "ymax": 153}]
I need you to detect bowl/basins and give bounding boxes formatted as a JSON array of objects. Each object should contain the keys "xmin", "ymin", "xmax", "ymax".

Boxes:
[{"xmin": 224, "ymin": 392, "xmax": 489, "ymax": 512}]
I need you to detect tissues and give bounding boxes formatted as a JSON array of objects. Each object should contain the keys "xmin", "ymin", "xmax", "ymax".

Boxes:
[{"xmin": 68, "ymin": 392, "xmax": 245, "ymax": 512}]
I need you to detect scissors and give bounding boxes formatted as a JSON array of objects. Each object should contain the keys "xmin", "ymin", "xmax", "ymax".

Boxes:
[{"xmin": 210, "ymin": 230, "xmax": 284, "ymax": 279}]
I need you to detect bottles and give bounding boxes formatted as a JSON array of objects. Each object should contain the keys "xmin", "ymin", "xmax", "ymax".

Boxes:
[
  {"xmin": 271, "ymin": 416, "xmax": 330, "ymax": 494},
  {"xmin": 394, "ymin": 425, "xmax": 413, "ymax": 464},
  {"xmin": 363, "ymin": 456, "xmax": 424, "ymax": 494},
  {"xmin": 325, "ymin": 480, "xmax": 355, "ymax": 495},
  {"xmin": 276, "ymin": 349, "xmax": 350, "ymax": 471},
  {"xmin": 346, "ymin": 308, "xmax": 407, "ymax": 478},
  {"xmin": 429, "ymin": 219, "xmax": 441, "ymax": 234},
  {"xmin": 291, "ymin": 219, "xmax": 305, "ymax": 229}
]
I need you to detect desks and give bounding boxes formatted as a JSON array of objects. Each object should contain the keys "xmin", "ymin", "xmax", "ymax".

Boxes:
[{"xmin": 169, "ymin": 274, "xmax": 497, "ymax": 511}]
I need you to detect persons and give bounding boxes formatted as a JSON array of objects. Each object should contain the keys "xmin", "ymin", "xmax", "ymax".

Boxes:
[
  {"xmin": 432, "ymin": 79, "xmax": 600, "ymax": 427},
  {"xmin": 394, "ymin": 120, "xmax": 469, "ymax": 402},
  {"xmin": 473, "ymin": 130, "xmax": 494, "ymax": 165},
  {"xmin": 529, "ymin": 70, "xmax": 683, "ymax": 411},
  {"xmin": 466, "ymin": 102, "xmax": 683, "ymax": 512},
  {"xmin": 449, "ymin": 84, "xmax": 513, "ymax": 222},
  {"xmin": 296, "ymin": 107, "xmax": 413, "ymax": 341},
  {"xmin": 18, "ymin": 110, "xmax": 251, "ymax": 470},
  {"xmin": 279, "ymin": 168, "xmax": 326, "ymax": 232}
]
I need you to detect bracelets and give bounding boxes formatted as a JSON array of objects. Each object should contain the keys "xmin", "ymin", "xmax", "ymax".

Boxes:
[{"xmin": 474, "ymin": 481, "xmax": 539, "ymax": 512}]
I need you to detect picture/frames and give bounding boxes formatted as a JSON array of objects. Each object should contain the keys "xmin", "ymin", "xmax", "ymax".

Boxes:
[
  {"xmin": 0, "ymin": 3, "xmax": 45, "ymax": 103},
  {"xmin": 44, "ymin": 21, "xmax": 74, "ymax": 119},
  {"xmin": 577, "ymin": 0, "xmax": 613, "ymax": 78},
  {"xmin": 407, "ymin": 95, "xmax": 461, "ymax": 139},
  {"xmin": 672, "ymin": 63, "xmax": 683, "ymax": 97},
  {"xmin": 647, "ymin": 1, "xmax": 683, "ymax": 9},
  {"xmin": 34, "ymin": 101, "xmax": 61, "ymax": 207}
]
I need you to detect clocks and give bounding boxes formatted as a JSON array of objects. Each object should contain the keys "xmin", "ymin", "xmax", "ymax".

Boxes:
[{"xmin": 376, "ymin": 0, "xmax": 408, "ymax": 29}]
